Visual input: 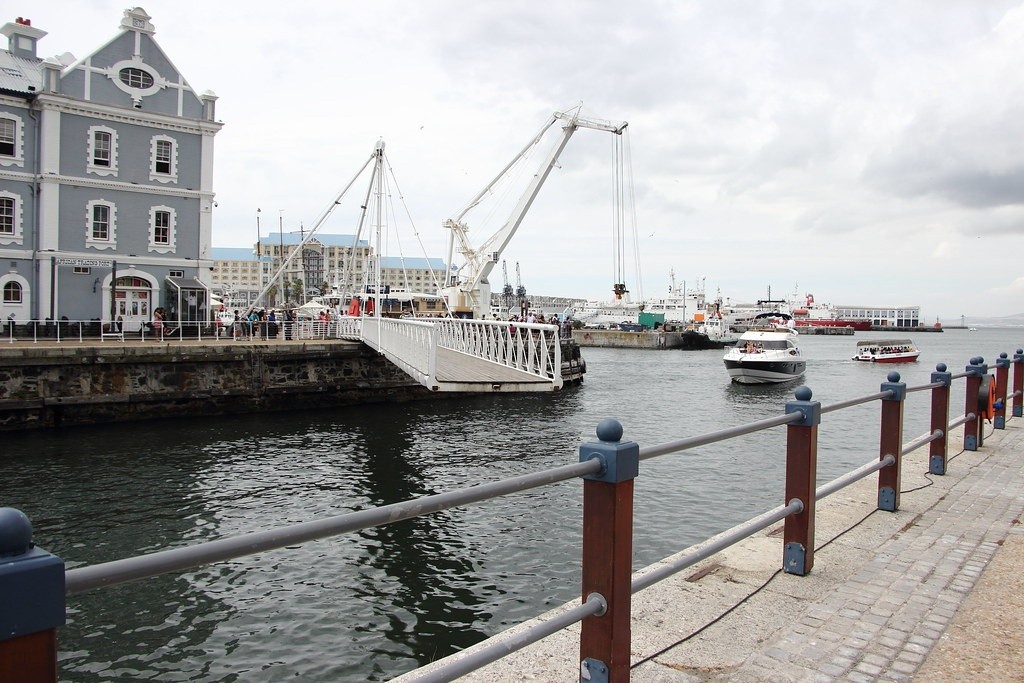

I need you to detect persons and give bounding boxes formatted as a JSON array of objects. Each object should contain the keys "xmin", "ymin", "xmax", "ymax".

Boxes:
[
  {"xmin": 219, "ymin": 305, "xmax": 559, "ymax": 340},
  {"xmin": 154, "ymin": 307, "xmax": 166, "ymax": 341},
  {"xmin": 859, "ymin": 345, "xmax": 910, "ymax": 354},
  {"xmin": 744, "ymin": 340, "xmax": 763, "ymax": 354}
]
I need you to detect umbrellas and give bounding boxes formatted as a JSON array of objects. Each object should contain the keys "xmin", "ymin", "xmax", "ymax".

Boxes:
[
  {"xmin": 210, "ymin": 293, "xmax": 223, "ymax": 299},
  {"xmin": 300, "ymin": 300, "xmax": 324, "ymax": 320},
  {"xmin": 210, "ymin": 298, "xmax": 224, "ymax": 306}
]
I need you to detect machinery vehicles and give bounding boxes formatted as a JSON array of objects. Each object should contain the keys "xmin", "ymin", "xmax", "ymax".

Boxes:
[{"xmin": 440, "ymin": 97, "xmax": 630, "ymax": 325}]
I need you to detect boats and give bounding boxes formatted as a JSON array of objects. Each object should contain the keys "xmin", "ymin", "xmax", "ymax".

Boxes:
[
  {"xmin": 570, "ymin": 267, "xmax": 944, "ymax": 348},
  {"xmin": 723, "ymin": 306, "xmax": 809, "ymax": 386},
  {"xmin": 851, "ymin": 337, "xmax": 923, "ymax": 364}
]
